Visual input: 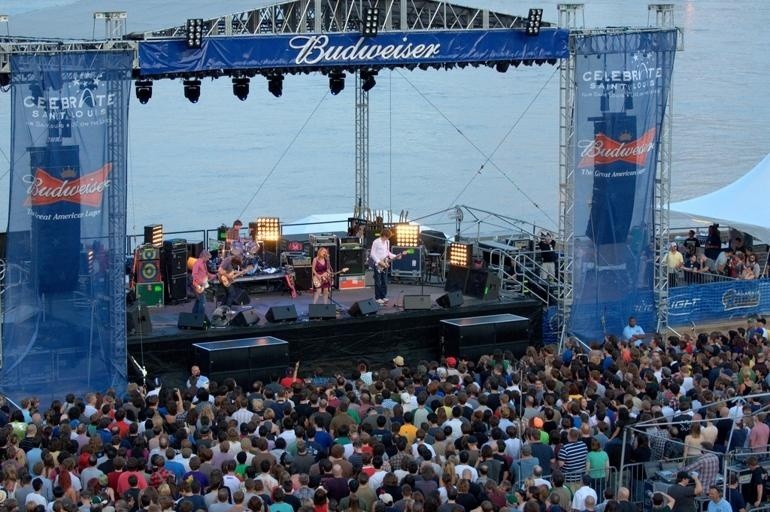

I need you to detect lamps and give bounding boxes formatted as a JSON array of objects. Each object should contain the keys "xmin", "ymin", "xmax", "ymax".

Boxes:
[
  {"xmin": 330, "ymin": 69, "xmax": 378, "ymax": 97},
  {"xmin": 133, "ymin": 68, "xmax": 285, "ymax": 105}
]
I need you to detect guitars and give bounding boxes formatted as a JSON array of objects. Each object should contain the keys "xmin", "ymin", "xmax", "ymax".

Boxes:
[
  {"xmin": 195, "ymin": 275, "xmax": 217, "ymax": 294},
  {"xmin": 221, "ymin": 269, "xmax": 249, "ymax": 287},
  {"xmin": 313, "ymin": 268, "xmax": 350, "ymax": 288},
  {"xmin": 375, "ymin": 250, "xmax": 407, "ymax": 274}
]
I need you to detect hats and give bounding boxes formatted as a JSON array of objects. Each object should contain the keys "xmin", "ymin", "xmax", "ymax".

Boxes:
[
  {"xmin": 393, "ymin": 356, "xmax": 405, "ymax": 366},
  {"xmin": 534, "ymin": 416, "xmax": 543, "ymax": 428},
  {"xmin": 447, "ymin": 357, "xmax": 456, "ymax": 367}
]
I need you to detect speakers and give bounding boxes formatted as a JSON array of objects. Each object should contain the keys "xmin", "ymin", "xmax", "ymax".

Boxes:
[
  {"xmin": 339, "ymin": 243, "xmax": 365, "ymax": 275},
  {"xmin": 28, "ymin": 151, "xmax": 81, "ymax": 296},
  {"xmin": 134, "ymin": 239, "xmax": 188, "ymax": 309},
  {"xmin": 293, "ymin": 266, "xmax": 314, "ymax": 291},
  {"xmin": 191, "ymin": 336, "xmax": 290, "ymax": 394},
  {"xmin": 585, "ymin": 115, "xmax": 637, "ymax": 246},
  {"xmin": 127, "ymin": 303, "xmax": 153, "ymax": 335},
  {"xmin": 440, "ymin": 313, "xmax": 530, "ymax": 362},
  {"xmin": 177, "ymin": 290, "xmax": 464, "ymax": 330},
  {"xmin": 444, "ymin": 265, "xmax": 501, "ymax": 300}
]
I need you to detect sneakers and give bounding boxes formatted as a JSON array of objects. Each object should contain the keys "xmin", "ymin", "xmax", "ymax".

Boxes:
[{"xmin": 376, "ymin": 298, "xmax": 389, "ymax": 304}]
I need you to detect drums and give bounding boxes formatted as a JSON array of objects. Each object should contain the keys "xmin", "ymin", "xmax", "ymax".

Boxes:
[{"xmin": 242, "ymin": 255, "xmax": 259, "ymax": 276}]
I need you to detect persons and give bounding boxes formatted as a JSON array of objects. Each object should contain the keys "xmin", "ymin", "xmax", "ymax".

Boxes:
[
  {"xmin": 191, "ymin": 250, "xmax": 221, "ymax": 313},
  {"xmin": 310, "ymin": 247, "xmax": 333, "ymax": 305},
  {"xmin": 537, "ymin": 231, "xmax": 557, "ymax": 281},
  {"xmin": 215, "ymin": 254, "xmax": 245, "ymax": 311},
  {"xmin": 370, "ymin": 228, "xmax": 403, "ymax": 305},
  {"xmin": 225, "ymin": 220, "xmax": 277, "ymax": 273},
  {"xmin": 667, "ymin": 224, "xmax": 760, "ymax": 285},
  {"xmin": 1, "ymin": 314, "xmax": 769, "ymax": 511}
]
